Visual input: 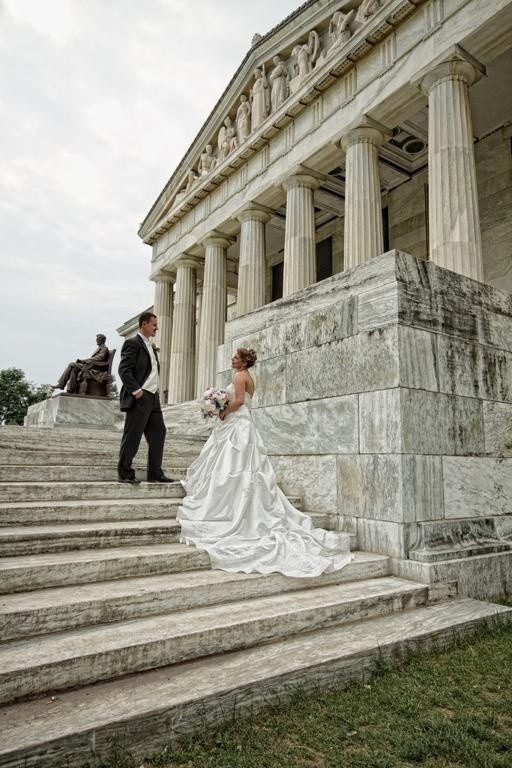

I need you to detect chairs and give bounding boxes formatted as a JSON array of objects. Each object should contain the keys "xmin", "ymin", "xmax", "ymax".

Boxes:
[{"xmin": 78, "ymin": 348, "xmax": 116, "ymax": 395}]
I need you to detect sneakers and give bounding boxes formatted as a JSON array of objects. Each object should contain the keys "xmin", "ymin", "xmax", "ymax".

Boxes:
[
  {"xmin": 118, "ymin": 474, "xmax": 142, "ymax": 485},
  {"xmin": 146, "ymin": 472, "xmax": 175, "ymax": 483}
]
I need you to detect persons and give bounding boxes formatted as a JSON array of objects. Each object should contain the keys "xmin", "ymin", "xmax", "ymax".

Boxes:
[
  {"xmin": 50, "ymin": 333, "xmax": 109, "ymax": 393},
  {"xmin": 117, "ymin": 311, "xmax": 174, "ymax": 485},
  {"xmin": 203, "ymin": 346, "xmax": 268, "ymax": 530}
]
